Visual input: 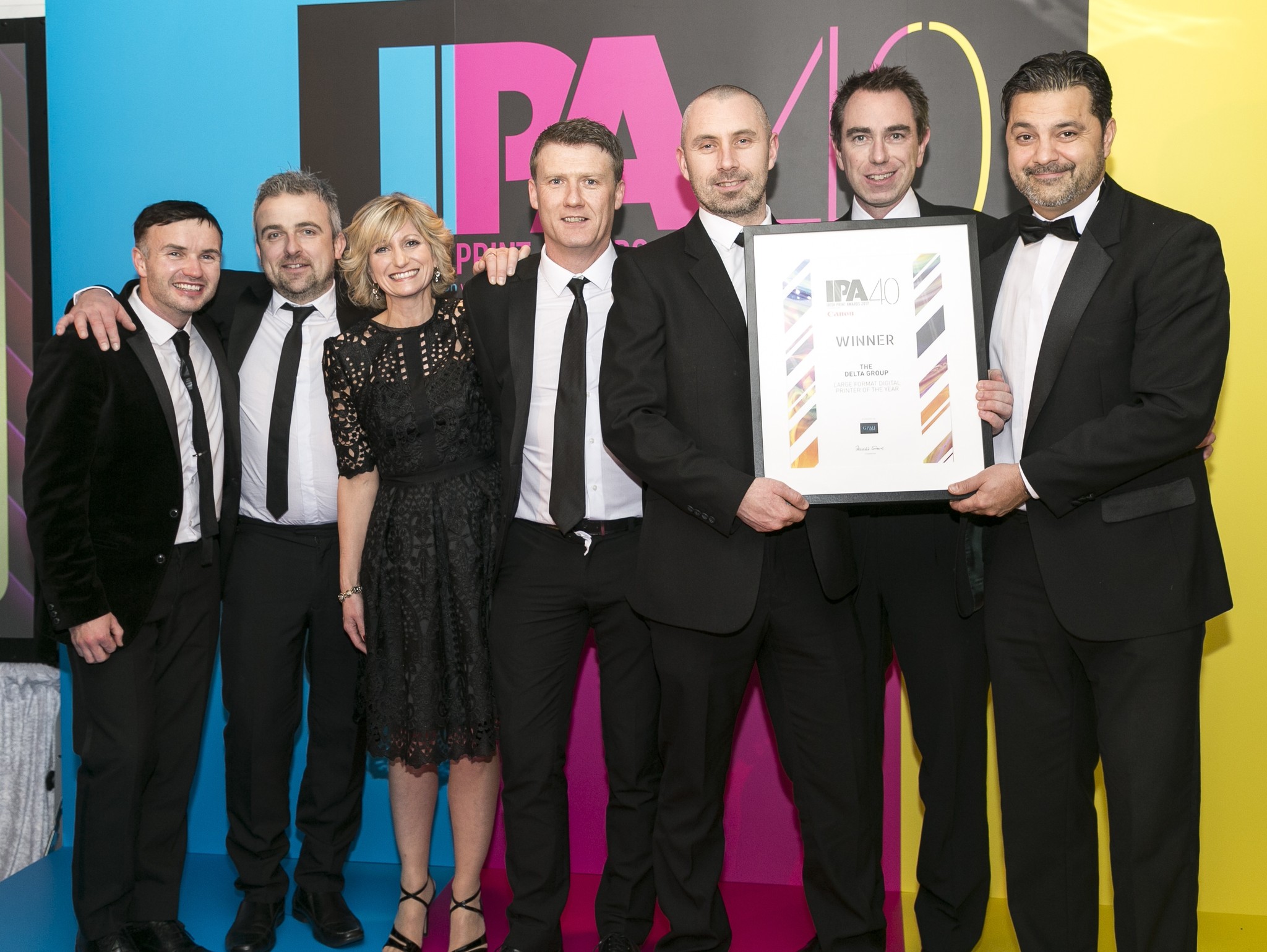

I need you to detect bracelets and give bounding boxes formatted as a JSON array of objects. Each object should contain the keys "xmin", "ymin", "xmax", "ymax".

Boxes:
[{"xmin": 337, "ymin": 586, "xmax": 362, "ymax": 603}]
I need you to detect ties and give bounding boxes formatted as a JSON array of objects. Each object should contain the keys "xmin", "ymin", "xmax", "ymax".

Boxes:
[
  {"xmin": 549, "ymin": 279, "xmax": 590, "ymax": 536},
  {"xmin": 171, "ymin": 332, "xmax": 220, "ymax": 564},
  {"xmin": 264, "ymin": 302, "xmax": 317, "ymax": 521}
]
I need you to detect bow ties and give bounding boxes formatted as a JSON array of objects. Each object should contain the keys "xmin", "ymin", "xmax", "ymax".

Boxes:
[{"xmin": 1016, "ymin": 213, "xmax": 1081, "ymax": 246}]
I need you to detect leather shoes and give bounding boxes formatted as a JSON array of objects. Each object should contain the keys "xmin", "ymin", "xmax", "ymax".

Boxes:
[
  {"xmin": 291, "ymin": 884, "xmax": 364, "ymax": 946},
  {"xmin": 598, "ymin": 933, "xmax": 638, "ymax": 952},
  {"xmin": 74, "ymin": 925, "xmax": 158, "ymax": 951},
  {"xmin": 128, "ymin": 919, "xmax": 212, "ymax": 952},
  {"xmin": 224, "ymin": 895, "xmax": 286, "ymax": 952}
]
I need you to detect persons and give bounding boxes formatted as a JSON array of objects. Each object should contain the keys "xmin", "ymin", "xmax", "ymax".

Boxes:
[
  {"xmin": 24, "ymin": 200, "xmax": 242, "ymax": 952},
  {"xmin": 466, "ymin": 117, "xmax": 667, "ymax": 952},
  {"xmin": 316, "ymin": 190, "xmax": 534, "ymax": 950},
  {"xmin": 594, "ymin": 82, "xmax": 1013, "ymax": 952},
  {"xmin": 793, "ymin": 69, "xmax": 1219, "ymax": 950},
  {"xmin": 958, "ymin": 49, "xmax": 1237, "ymax": 952},
  {"xmin": 49, "ymin": 171, "xmax": 536, "ymax": 952}
]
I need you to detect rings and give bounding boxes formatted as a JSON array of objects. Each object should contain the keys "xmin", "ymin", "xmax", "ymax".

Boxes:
[{"xmin": 485, "ymin": 252, "xmax": 498, "ymax": 257}]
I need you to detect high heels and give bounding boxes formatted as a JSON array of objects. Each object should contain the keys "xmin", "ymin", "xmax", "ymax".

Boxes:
[
  {"xmin": 384, "ymin": 869, "xmax": 438, "ymax": 952},
  {"xmin": 447, "ymin": 883, "xmax": 487, "ymax": 952}
]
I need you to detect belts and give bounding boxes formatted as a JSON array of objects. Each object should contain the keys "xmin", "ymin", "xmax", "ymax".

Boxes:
[
  {"xmin": 239, "ymin": 524, "xmax": 341, "ymax": 547},
  {"xmin": 514, "ymin": 516, "xmax": 642, "ymax": 536}
]
